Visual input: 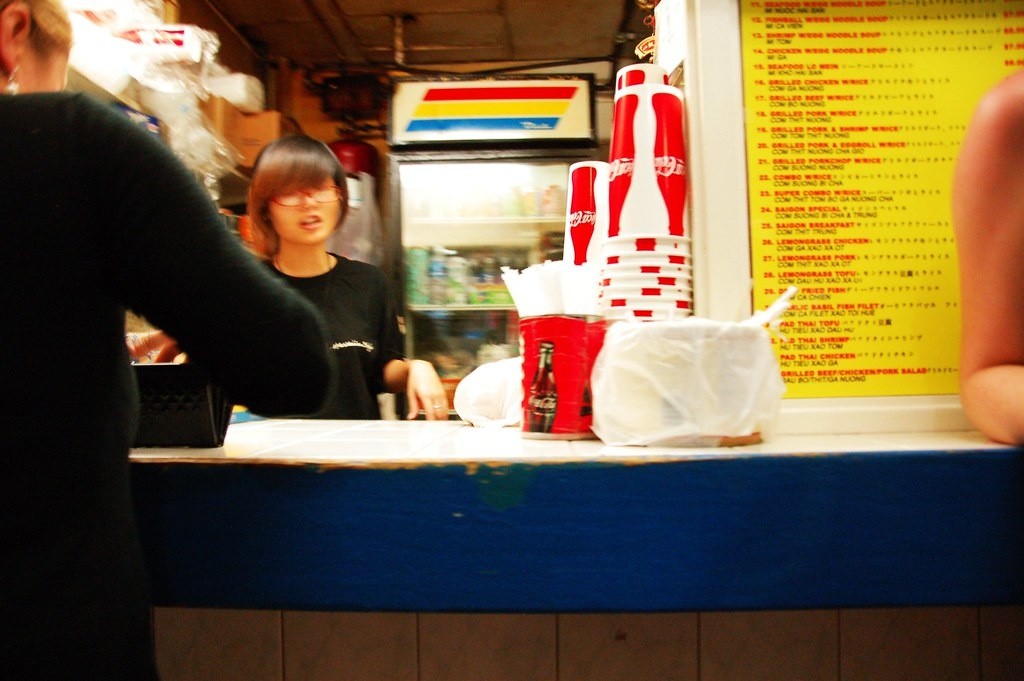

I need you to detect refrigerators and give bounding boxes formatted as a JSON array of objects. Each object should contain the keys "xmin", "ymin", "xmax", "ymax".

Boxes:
[{"xmin": 386, "ymin": 74, "xmax": 597, "ymax": 419}]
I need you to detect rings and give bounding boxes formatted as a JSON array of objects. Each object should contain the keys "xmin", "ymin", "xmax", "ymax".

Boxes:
[{"xmin": 433, "ymin": 404, "xmax": 444, "ymax": 409}]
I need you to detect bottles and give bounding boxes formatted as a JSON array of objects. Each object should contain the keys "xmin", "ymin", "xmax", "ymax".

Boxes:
[
  {"xmin": 528, "ymin": 342, "xmax": 556, "ymax": 433},
  {"xmin": 404, "ymin": 181, "xmax": 562, "ymax": 409}
]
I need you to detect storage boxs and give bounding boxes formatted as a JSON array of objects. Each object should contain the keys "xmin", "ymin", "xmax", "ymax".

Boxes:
[{"xmin": 201, "ymin": 97, "xmax": 297, "ymax": 175}]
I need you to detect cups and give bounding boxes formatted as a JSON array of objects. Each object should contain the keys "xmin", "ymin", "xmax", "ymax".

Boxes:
[
  {"xmin": 517, "ymin": 316, "xmax": 605, "ymax": 439},
  {"xmin": 563, "ymin": 61, "xmax": 693, "ymax": 324}
]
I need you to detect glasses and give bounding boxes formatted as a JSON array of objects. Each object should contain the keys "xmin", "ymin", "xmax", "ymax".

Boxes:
[{"xmin": 268, "ymin": 185, "xmax": 344, "ymax": 207}]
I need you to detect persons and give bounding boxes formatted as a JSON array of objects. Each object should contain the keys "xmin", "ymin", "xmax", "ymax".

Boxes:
[
  {"xmin": 950, "ymin": 67, "xmax": 1024, "ymax": 444},
  {"xmin": 0, "ymin": 0, "xmax": 341, "ymax": 681},
  {"xmin": 246, "ymin": 134, "xmax": 450, "ymax": 421}
]
